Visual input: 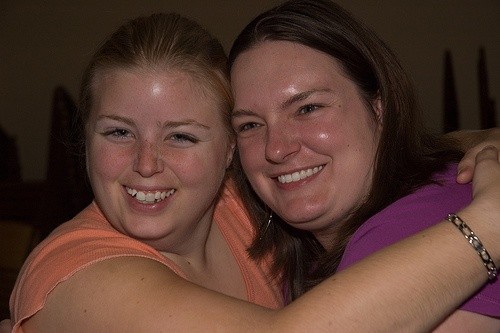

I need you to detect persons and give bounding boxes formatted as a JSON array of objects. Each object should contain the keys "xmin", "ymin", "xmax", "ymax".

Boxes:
[
  {"xmin": 10, "ymin": 12, "xmax": 499, "ymax": 333},
  {"xmin": 1, "ymin": 0, "xmax": 499, "ymax": 333}
]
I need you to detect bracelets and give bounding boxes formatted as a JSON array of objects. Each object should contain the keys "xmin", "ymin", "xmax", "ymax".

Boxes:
[{"xmin": 448, "ymin": 212, "xmax": 498, "ymax": 280}]
